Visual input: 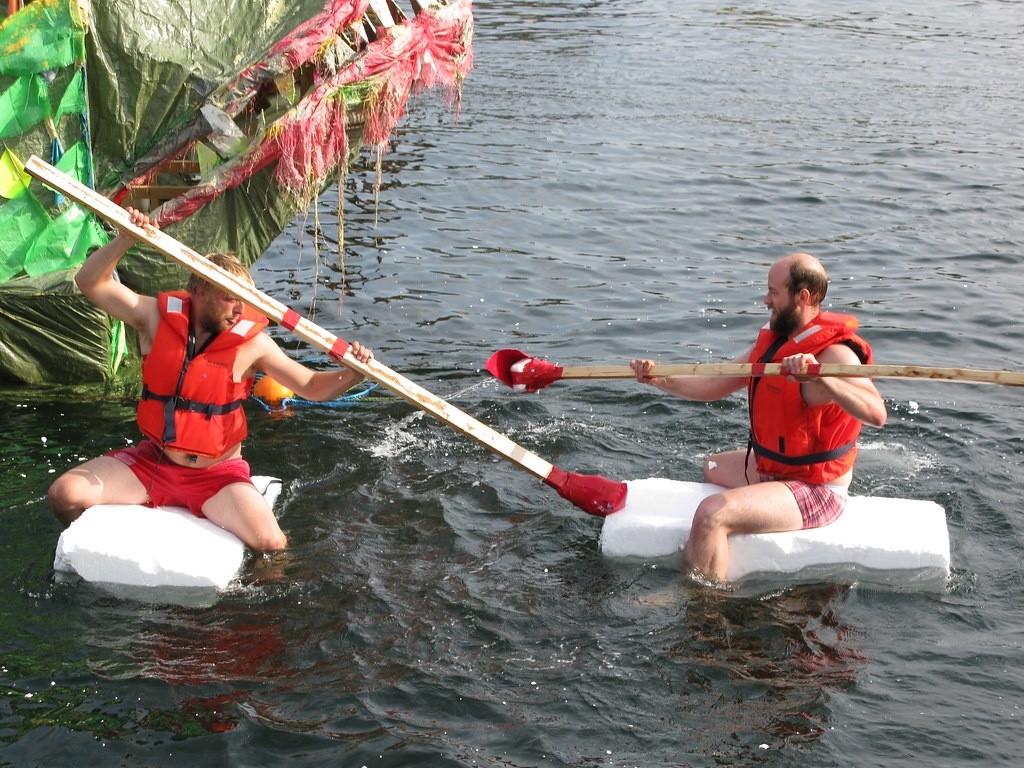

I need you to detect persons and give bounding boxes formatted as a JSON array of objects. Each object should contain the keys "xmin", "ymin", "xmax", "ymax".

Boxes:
[
  {"xmin": 48, "ymin": 206, "xmax": 372, "ymax": 550},
  {"xmin": 629, "ymin": 254, "xmax": 886, "ymax": 581}
]
